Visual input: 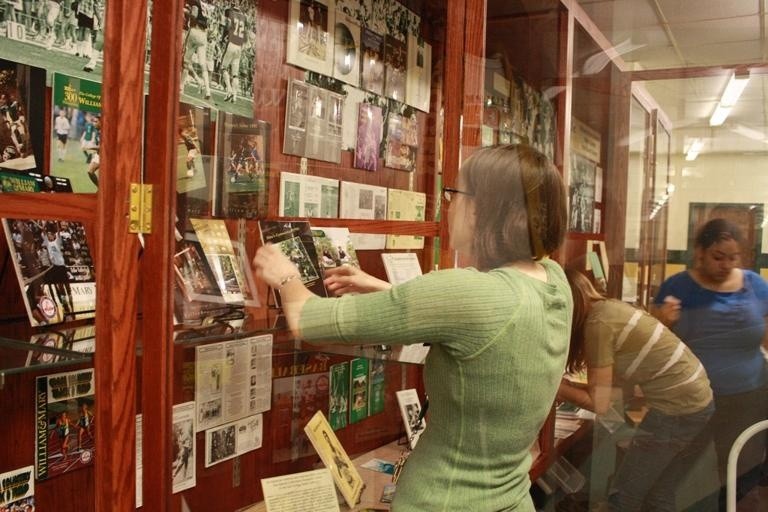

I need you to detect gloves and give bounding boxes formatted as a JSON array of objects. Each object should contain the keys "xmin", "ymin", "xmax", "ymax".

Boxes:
[{"xmin": 189, "ymin": 15, "xmax": 197, "ymax": 27}]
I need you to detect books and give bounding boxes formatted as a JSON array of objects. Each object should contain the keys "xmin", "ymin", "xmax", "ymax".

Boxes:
[{"xmin": 0, "ymin": 0, "xmax": 610, "ymax": 512}]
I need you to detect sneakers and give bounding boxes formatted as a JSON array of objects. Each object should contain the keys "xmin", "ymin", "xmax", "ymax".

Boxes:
[
  {"xmin": 197, "ymin": 84, "xmax": 211, "ymax": 100},
  {"xmin": 84, "ymin": 62, "xmax": 95, "ymax": 72},
  {"xmin": 223, "ymin": 90, "xmax": 236, "ymax": 103}
]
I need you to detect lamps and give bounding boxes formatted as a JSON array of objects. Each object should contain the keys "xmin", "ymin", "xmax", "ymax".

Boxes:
[{"xmin": 683, "ymin": 66, "xmax": 750, "ymax": 163}]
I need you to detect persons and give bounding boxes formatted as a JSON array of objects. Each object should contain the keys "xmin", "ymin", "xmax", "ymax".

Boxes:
[
  {"xmin": 556, "ymin": 267, "xmax": 717, "ymax": 509},
  {"xmin": 646, "ymin": 214, "xmax": 768, "ymax": 512}
]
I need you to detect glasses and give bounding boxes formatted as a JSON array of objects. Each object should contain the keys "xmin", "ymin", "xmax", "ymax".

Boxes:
[{"xmin": 442, "ymin": 185, "xmax": 474, "ymax": 203}]
